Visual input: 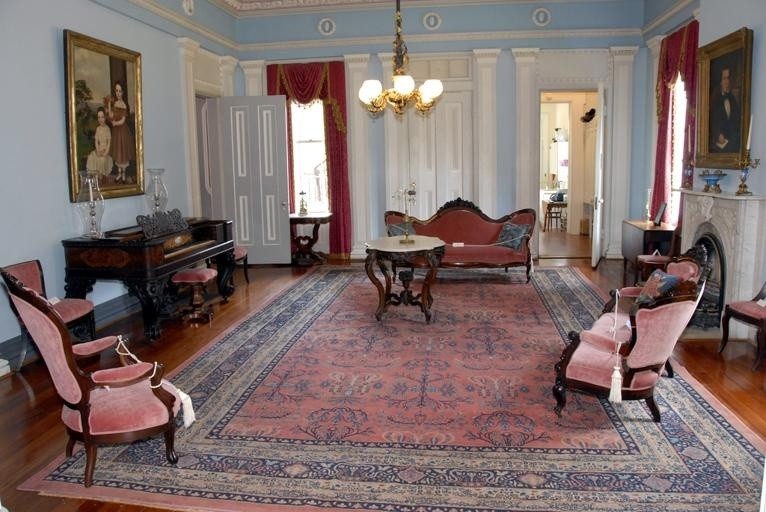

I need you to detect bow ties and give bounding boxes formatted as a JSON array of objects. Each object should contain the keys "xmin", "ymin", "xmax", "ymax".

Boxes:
[{"xmin": 721, "ymin": 92, "xmax": 732, "ymax": 101}]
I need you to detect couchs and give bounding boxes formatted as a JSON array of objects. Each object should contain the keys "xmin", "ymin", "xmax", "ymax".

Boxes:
[{"xmin": 381, "ymin": 194, "xmax": 538, "ymax": 287}]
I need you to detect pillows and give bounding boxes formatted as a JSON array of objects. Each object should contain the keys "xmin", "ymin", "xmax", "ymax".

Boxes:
[
  {"xmin": 496, "ymin": 220, "xmax": 532, "ymax": 252},
  {"xmin": 383, "ymin": 220, "xmax": 417, "ymax": 238}
]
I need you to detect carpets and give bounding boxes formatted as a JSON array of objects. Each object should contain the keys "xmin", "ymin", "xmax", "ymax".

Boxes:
[{"xmin": 12, "ymin": 263, "xmax": 766, "ymax": 511}]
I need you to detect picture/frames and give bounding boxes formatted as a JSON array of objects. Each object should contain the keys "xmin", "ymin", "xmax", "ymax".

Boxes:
[
  {"xmin": 60, "ymin": 24, "xmax": 147, "ymax": 205},
  {"xmin": 654, "ymin": 202, "xmax": 667, "ymax": 226},
  {"xmin": 693, "ymin": 24, "xmax": 756, "ymax": 174}
]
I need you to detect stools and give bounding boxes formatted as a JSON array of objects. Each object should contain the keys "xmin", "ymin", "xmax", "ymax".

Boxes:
[{"xmin": 168, "ymin": 265, "xmax": 218, "ymax": 326}]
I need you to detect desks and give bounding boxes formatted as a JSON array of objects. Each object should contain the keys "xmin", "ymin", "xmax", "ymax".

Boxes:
[
  {"xmin": 362, "ymin": 232, "xmax": 447, "ymax": 326},
  {"xmin": 540, "ymin": 200, "xmax": 570, "ymax": 234},
  {"xmin": 287, "ymin": 212, "xmax": 335, "ymax": 269}
]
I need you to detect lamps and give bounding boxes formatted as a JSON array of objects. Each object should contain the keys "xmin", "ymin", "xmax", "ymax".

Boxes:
[
  {"xmin": 297, "ymin": 186, "xmax": 309, "ymax": 217},
  {"xmin": 355, "ymin": 0, "xmax": 447, "ymax": 122},
  {"xmin": 389, "ymin": 180, "xmax": 419, "ymax": 245}
]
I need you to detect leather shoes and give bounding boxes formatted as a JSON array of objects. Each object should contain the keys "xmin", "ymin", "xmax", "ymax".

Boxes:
[
  {"xmin": 114, "ymin": 173, "xmax": 121, "ymax": 182},
  {"xmin": 121, "ymin": 174, "xmax": 127, "ymax": 184}
]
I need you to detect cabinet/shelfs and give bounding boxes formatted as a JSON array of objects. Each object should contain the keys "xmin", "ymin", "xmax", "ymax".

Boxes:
[{"xmin": 621, "ymin": 219, "xmax": 678, "ymax": 290}]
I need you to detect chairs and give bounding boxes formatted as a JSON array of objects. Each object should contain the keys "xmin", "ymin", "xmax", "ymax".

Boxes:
[
  {"xmin": 1, "ymin": 258, "xmax": 99, "ymax": 375},
  {"xmin": 546, "ymin": 203, "xmax": 565, "ymax": 234},
  {"xmin": 548, "ymin": 249, "xmax": 766, "ymax": 426},
  {"xmin": 1, "ymin": 264, "xmax": 195, "ymax": 492},
  {"xmin": 231, "ymin": 240, "xmax": 250, "ymax": 287}
]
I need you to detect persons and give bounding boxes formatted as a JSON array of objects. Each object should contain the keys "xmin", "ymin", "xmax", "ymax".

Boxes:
[
  {"xmin": 710, "ymin": 65, "xmax": 740, "ymax": 154},
  {"xmin": 85, "ymin": 106, "xmax": 114, "ymax": 189},
  {"xmin": 107, "ymin": 80, "xmax": 136, "ymax": 184}
]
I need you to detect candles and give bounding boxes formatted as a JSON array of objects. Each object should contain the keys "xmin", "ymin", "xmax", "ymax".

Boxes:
[
  {"xmin": 747, "ymin": 114, "xmax": 754, "ymax": 151},
  {"xmin": 688, "ymin": 124, "xmax": 692, "ymax": 154}
]
